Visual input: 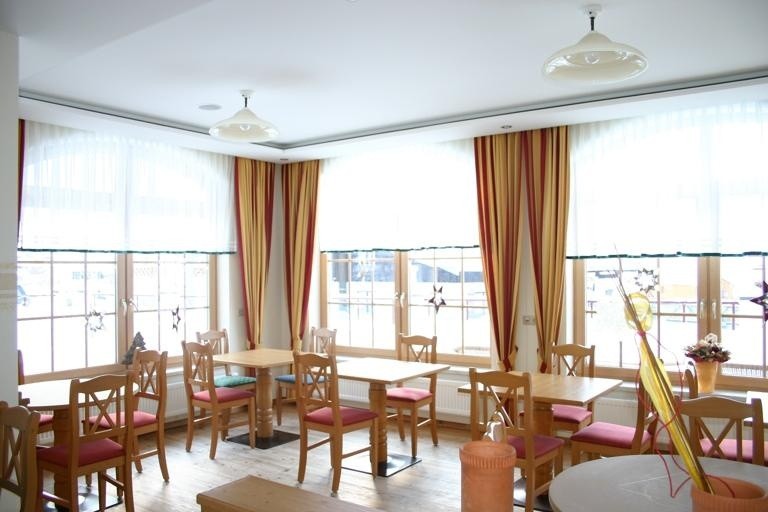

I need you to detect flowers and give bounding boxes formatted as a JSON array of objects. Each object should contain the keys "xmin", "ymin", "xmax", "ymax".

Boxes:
[{"xmin": 684, "ymin": 333, "xmax": 735, "ymax": 361}]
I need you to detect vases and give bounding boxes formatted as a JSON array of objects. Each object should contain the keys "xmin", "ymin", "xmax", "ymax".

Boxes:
[{"xmin": 693, "ymin": 357, "xmax": 720, "ymax": 393}]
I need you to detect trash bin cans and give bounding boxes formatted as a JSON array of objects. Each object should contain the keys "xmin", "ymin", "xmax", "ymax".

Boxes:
[
  {"xmin": 459, "ymin": 441, "xmax": 517, "ymax": 512},
  {"xmin": 691, "ymin": 476, "xmax": 768, "ymax": 512}
]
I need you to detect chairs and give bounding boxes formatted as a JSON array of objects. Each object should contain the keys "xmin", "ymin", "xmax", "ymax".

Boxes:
[
  {"xmin": 196, "ymin": 328, "xmax": 256, "ymax": 442},
  {"xmin": 273, "ymin": 327, "xmax": 337, "ymax": 426},
  {"xmin": 669, "ymin": 393, "xmax": 765, "ymax": 468},
  {"xmin": 467, "ymin": 367, "xmax": 565, "ymax": 512},
  {"xmin": 181, "ymin": 340, "xmax": 256, "ymax": 460},
  {"xmin": 291, "ymin": 350, "xmax": 380, "ymax": 498},
  {"xmin": 386, "ymin": 332, "xmax": 440, "ymax": 458},
  {"xmin": 1, "ymin": 399, "xmax": 42, "ymax": 512},
  {"xmin": 567, "ymin": 358, "xmax": 668, "ymax": 469},
  {"xmin": 519, "ymin": 341, "xmax": 598, "ymax": 481},
  {"xmin": 81, "ymin": 346, "xmax": 170, "ymax": 485},
  {"xmin": 36, "ymin": 372, "xmax": 135, "ymax": 512},
  {"xmin": 682, "ymin": 369, "xmax": 767, "ymax": 464}
]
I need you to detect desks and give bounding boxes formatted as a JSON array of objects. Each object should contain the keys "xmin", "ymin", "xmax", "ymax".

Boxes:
[
  {"xmin": 548, "ymin": 452, "xmax": 768, "ymax": 512},
  {"xmin": 199, "ymin": 348, "xmax": 315, "ymax": 450},
  {"xmin": 457, "ymin": 369, "xmax": 628, "ymax": 497},
  {"xmin": 18, "ymin": 376, "xmax": 140, "ymax": 488},
  {"xmin": 303, "ymin": 356, "xmax": 450, "ymax": 479},
  {"xmin": 743, "ymin": 389, "xmax": 768, "ymax": 426}
]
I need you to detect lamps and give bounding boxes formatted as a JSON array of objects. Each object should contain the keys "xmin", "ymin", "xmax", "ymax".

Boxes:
[
  {"xmin": 207, "ymin": 87, "xmax": 275, "ymax": 149},
  {"xmin": 541, "ymin": 4, "xmax": 651, "ymax": 90}
]
